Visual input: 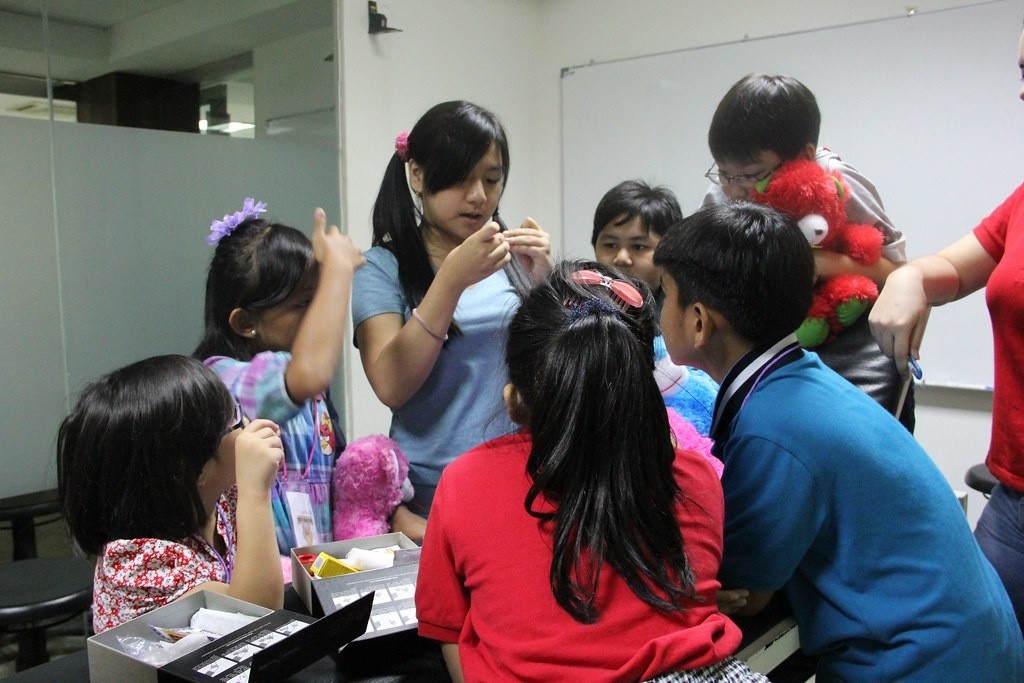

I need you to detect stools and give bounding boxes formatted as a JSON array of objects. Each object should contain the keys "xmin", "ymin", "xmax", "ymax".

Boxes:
[{"xmin": 0, "ymin": 557, "xmax": 96, "ymax": 672}]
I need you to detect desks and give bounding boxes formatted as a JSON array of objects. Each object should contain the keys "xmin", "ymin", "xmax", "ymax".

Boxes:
[{"xmin": 0, "ymin": 591, "xmax": 802, "ymax": 683}]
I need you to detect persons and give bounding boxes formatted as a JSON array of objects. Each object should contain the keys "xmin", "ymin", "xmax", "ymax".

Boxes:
[
  {"xmin": 54, "ymin": 100, "xmax": 557, "ymax": 638},
  {"xmin": 411, "ymin": 29, "xmax": 1024, "ymax": 683}
]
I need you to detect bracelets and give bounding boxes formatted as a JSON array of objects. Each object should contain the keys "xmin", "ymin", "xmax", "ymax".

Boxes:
[{"xmin": 412, "ymin": 308, "xmax": 448, "ymax": 341}]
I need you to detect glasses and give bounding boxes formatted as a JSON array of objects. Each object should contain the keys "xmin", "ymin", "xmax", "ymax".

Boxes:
[
  {"xmin": 705, "ymin": 161, "xmax": 783, "ymax": 189},
  {"xmin": 220, "ymin": 394, "xmax": 251, "ymax": 437}
]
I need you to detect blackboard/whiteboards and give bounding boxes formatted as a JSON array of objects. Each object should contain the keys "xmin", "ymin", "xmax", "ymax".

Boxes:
[{"xmin": 560, "ymin": 2, "xmax": 1016, "ymax": 391}]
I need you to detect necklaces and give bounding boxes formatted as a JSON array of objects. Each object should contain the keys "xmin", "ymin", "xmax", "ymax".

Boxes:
[{"xmin": 194, "ymin": 501, "xmax": 232, "ymax": 585}]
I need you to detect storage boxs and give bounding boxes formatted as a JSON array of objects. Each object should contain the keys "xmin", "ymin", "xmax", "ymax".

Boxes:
[
  {"xmin": 87, "ymin": 589, "xmax": 376, "ymax": 683},
  {"xmin": 290, "ymin": 531, "xmax": 420, "ymax": 657}
]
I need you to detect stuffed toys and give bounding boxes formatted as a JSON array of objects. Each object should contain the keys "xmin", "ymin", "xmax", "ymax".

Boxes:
[
  {"xmin": 650, "ymin": 334, "xmax": 724, "ymax": 482},
  {"xmin": 330, "ymin": 432, "xmax": 416, "ymax": 541},
  {"xmin": 746, "ymin": 158, "xmax": 884, "ymax": 351}
]
